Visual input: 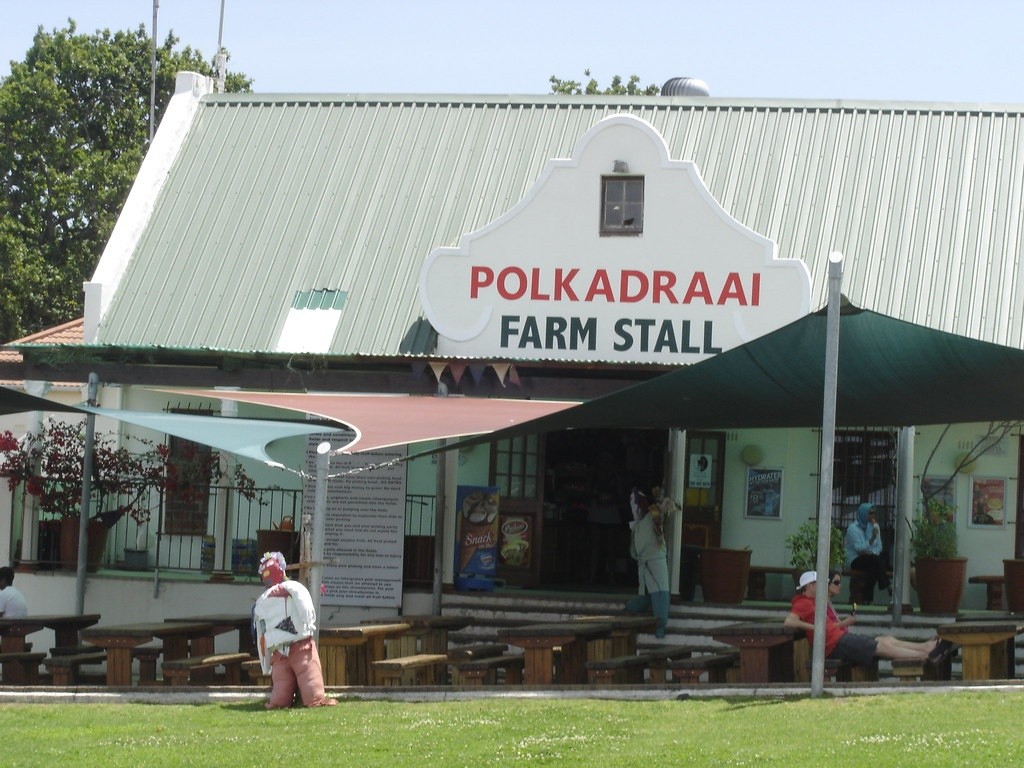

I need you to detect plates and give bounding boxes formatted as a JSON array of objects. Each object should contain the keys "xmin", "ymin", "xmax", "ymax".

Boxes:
[{"xmin": 972, "ymin": 498, "xmax": 1003, "ymax": 524}]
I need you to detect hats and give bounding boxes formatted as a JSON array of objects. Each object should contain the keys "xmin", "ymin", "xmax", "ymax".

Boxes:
[{"xmin": 796, "ymin": 571, "xmax": 817, "ymax": 590}]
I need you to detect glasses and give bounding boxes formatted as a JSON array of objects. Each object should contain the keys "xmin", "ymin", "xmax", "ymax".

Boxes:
[
  {"xmin": 870, "ymin": 511, "xmax": 876, "ymax": 515},
  {"xmin": 832, "ymin": 580, "xmax": 840, "ymax": 585}
]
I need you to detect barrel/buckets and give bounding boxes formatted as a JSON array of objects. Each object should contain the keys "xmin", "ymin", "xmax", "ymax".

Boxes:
[{"xmin": 699, "ymin": 548, "xmax": 753, "ymax": 605}]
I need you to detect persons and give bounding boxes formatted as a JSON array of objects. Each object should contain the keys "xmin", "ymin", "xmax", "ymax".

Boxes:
[
  {"xmin": 253, "ymin": 552, "xmax": 338, "ymax": 708},
  {"xmin": 629, "ymin": 487, "xmax": 678, "ymax": 639},
  {"xmin": 844, "ymin": 502, "xmax": 892, "ymax": 604},
  {"xmin": 0, "ymin": 567, "xmax": 28, "ymax": 619},
  {"xmin": 785, "ymin": 570, "xmax": 956, "ymax": 662}
]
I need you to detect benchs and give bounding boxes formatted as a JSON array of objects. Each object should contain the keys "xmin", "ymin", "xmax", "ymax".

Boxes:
[
  {"xmin": 748, "ymin": 566, "xmax": 893, "ymax": 606},
  {"xmin": 891, "ymin": 645, "xmax": 958, "ymax": 681},
  {"xmin": 0, "ymin": 646, "xmax": 879, "ymax": 686},
  {"xmin": 969, "ymin": 575, "xmax": 1006, "ymax": 611}
]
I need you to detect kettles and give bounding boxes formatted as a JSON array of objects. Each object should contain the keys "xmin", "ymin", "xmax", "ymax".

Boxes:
[{"xmin": 273, "ymin": 517, "xmax": 294, "ymax": 531}]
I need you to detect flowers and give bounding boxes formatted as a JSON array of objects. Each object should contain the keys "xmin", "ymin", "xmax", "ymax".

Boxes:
[{"xmin": 0, "ymin": 417, "xmax": 270, "ymax": 524}]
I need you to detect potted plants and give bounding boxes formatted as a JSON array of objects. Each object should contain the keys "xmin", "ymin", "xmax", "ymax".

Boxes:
[
  {"xmin": 786, "ymin": 517, "xmax": 848, "ymax": 587},
  {"xmin": 909, "ymin": 495, "xmax": 968, "ymax": 613}
]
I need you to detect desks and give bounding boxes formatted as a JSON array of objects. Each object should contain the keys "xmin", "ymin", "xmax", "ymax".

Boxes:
[
  {"xmin": 936, "ymin": 619, "xmax": 1024, "ymax": 679},
  {"xmin": 0, "ymin": 612, "xmax": 810, "ymax": 686}
]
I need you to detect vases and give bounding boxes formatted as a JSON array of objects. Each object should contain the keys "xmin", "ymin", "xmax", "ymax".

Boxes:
[
  {"xmin": 1003, "ymin": 560, "xmax": 1024, "ymax": 612},
  {"xmin": 256, "ymin": 530, "xmax": 301, "ymax": 573},
  {"xmin": 60, "ymin": 518, "xmax": 108, "ymax": 574},
  {"xmin": 702, "ymin": 547, "xmax": 752, "ymax": 604},
  {"xmin": 125, "ymin": 548, "xmax": 149, "ymax": 571}
]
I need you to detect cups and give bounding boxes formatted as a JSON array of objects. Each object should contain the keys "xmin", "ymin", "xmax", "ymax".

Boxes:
[{"xmin": 500, "ymin": 520, "xmax": 529, "ymax": 567}]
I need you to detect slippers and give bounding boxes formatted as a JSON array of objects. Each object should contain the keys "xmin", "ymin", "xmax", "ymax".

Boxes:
[
  {"xmin": 928, "ymin": 651, "xmax": 946, "ymax": 665},
  {"xmin": 929, "ymin": 637, "xmax": 951, "ymax": 658}
]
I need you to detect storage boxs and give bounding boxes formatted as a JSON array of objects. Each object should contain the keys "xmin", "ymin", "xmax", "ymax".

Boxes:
[{"xmin": 202, "ymin": 536, "xmax": 256, "ymax": 575}]
